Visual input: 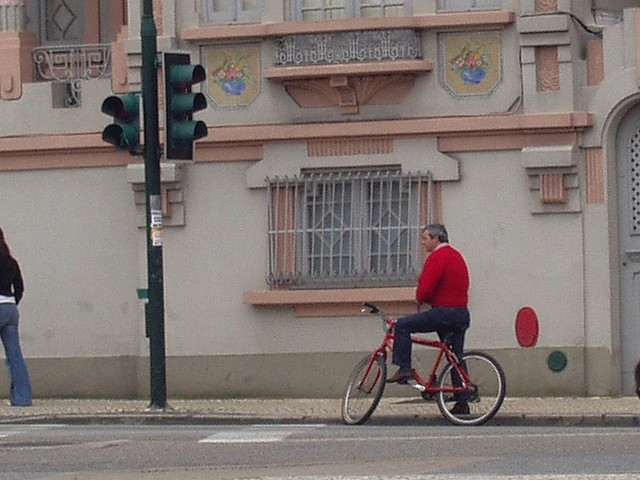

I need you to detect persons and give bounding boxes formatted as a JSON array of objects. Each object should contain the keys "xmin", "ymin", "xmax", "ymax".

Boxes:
[
  {"xmin": 386, "ymin": 224, "xmax": 470, "ymax": 415},
  {"xmin": 0, "ymin": 228, "xmax": 32, "ymax": 406}
]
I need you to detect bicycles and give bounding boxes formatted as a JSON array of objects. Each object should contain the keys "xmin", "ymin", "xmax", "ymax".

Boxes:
[{"xmin": 342, "ymin": 299, "xmax": 506, "ymax": 426}]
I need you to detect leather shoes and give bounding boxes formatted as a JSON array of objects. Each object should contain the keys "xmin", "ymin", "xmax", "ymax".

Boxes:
[
  {"xmin": 386, "ymin": 368, "xmax": 417, "ymax": 383},
  {"xmin": 449, "ymin": 407, "xmax": 470, "ymax": 414}
]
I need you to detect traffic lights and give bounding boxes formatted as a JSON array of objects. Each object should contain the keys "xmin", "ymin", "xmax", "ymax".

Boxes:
[
  {"xmin": 101, "ymin": 96, "xmax": 141, "ymax": 155},
  {"xmin": 162, "ymin": 48, "xmax": 208, "ymax": 161}
]
